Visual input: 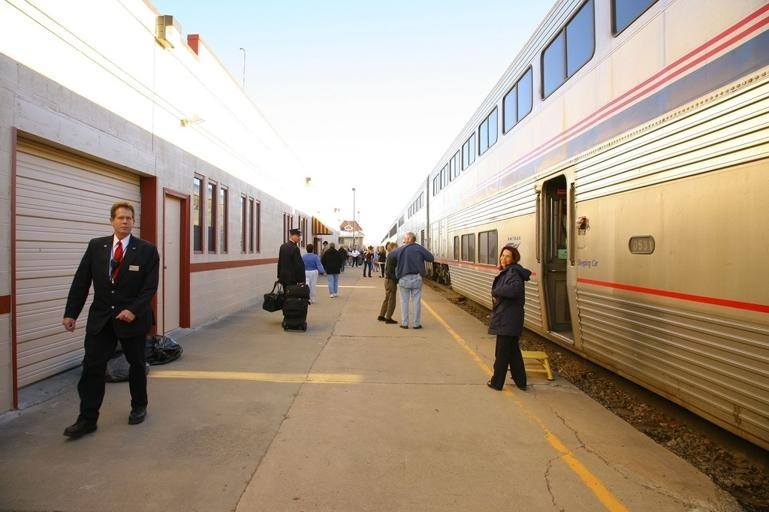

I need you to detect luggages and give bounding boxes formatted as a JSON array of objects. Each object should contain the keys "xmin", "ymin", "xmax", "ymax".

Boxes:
[{"xmin": 282, "ymin": 283, "xmax": 309, "ymax": 329}]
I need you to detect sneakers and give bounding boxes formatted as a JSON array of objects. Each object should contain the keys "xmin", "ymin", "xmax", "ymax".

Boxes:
[
  {"xmin": 489, "ymin": 380, "xmax": 495, "ymax": 387},
  {"xmin": 377, "ymin": 316, "xmax": 422, "ymax": 329},
  {"xmin": 329, "ymin": 293, "xmax": 337, "ymax": 298}
]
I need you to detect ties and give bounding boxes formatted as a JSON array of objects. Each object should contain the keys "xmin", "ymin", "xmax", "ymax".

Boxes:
[{"xmin": 112, "ymin": 242, "xmax": 123, "ymax": 278}]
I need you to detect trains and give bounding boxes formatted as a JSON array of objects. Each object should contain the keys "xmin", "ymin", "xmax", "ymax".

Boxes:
[{"xmin": 379, "ymin": 0, "xmax": 768, "ymax": 452}]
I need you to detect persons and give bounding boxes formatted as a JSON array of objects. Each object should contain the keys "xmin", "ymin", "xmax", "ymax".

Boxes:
[
  {"xmin": 277, "ymin": 229, "xmax": 434, "ymax": 329},
  {"xmin": 487, "ymin": 246, "xmax": 531, "ymax": 390},
  {"xmin": 63, "ymin": 201, "xmax": 159, "ymax": 436}
]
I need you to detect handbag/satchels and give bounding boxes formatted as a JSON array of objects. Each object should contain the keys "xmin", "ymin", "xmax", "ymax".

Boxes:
[{"xmin": 263, "ymin": 282, "xmax": 284, "ymax": 311}]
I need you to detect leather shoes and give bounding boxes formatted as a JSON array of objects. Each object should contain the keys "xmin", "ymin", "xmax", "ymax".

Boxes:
[
  {"xmin": 64, "ymin": 416, "xmax": 97, "ymax": 436},
  {"xmin": 128, "ymin": 406, "xmax": 145, "ymax": 423}
]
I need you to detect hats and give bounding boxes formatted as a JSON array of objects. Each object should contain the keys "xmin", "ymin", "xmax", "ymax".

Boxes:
[{"xmin": 290, "ymin": 228, "xmax": 301, "ymax": 234}]
